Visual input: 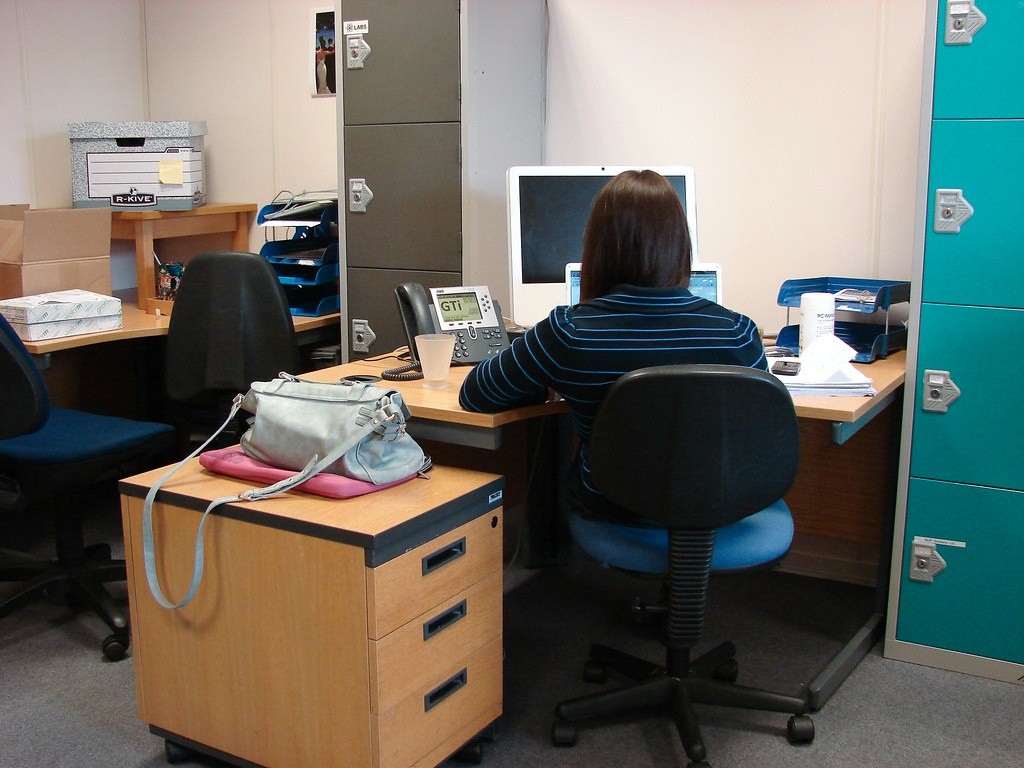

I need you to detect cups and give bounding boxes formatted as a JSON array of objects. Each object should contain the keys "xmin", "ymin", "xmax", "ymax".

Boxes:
[
  {"xmin": 414, "ymin": 334, "xmax": 456, "ymax": 390},
  {"xmin": 159, "ymin": 261, "xmax": 185, "ymax": 301}
]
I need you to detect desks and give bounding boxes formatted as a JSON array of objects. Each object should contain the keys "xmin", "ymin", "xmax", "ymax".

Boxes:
[
  {"xmin": 291, "ymin": 320, "xmax": 907, "ymax": 712},
  {"xmin": 22, "ymin": 204, "xmax": 341, "ymax": 354}
]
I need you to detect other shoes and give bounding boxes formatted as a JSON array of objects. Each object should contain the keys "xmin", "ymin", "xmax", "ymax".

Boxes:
[{"xmin": 618, "ymin": 590, "xmax": 670, "ymax": 640}]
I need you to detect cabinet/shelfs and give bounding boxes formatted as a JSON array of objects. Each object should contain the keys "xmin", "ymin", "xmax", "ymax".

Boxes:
[
  {"xmin": 259, "ymin": 204, "xmax": 341, "ymax": 317},
  {"xmin": 776, "ymin": 277, "xmax": 910, "ymax": 363},
  {"xmin": 118, "ymin": 444, "xmax": 505, "ymax": 768},
  {"xmin": 883, "ymin": 0, "xmax": 1024, "ymax": 685},
  {"xmin": 335, "ymin": 0, "xmax": 551, "ymax": 364}
]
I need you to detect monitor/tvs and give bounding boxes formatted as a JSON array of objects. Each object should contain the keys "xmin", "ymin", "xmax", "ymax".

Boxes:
[{"xmin": 505, "ymin": 165, "xmax": 698, "ymax": 328}]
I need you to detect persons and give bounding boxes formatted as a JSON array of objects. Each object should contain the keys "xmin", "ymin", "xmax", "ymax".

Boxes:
[
  {"xmin": 316, "ymin": 36, "xmax": 336, "ymax": 94},
  {"xmin": 459, "ymin": 170, "xmax": 769, "ymax": 636}
]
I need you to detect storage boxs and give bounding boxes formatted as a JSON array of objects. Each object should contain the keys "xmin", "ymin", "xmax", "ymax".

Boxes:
[
  {"xmin": 0, "ymin": 204, "xmax": 112, "ymax": 297},
  {"xmin": 67, "ymin": 120, "xmax": 208, "ymax": 211},
  {"xmin": 0, "ymin": 289, "xmax": 123, "ymax": 341}
]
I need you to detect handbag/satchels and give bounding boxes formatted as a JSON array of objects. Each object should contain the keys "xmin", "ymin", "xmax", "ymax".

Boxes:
[{"xmin": 233, "ymin": 371, "xmax": 424, "ymax": 485}]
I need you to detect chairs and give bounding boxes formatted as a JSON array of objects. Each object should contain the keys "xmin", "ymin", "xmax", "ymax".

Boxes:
[
  {"xmin": 0, "ymin": 309, "xmax": 177, "ymax": 660},
  {"xmin": 165, "ymin": 252, "xmax": 300, "ymax": 460},
  {"xmin": 551, "ymin": 365, "xmax": 814, "ymax": 768}
]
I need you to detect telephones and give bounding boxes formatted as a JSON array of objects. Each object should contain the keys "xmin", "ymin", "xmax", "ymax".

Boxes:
[{"xmin": 394, "ymin": 283, "xmax": 510, "ymax": 372}]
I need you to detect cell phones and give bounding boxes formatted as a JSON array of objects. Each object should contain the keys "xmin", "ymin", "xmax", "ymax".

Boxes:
[{"xmin": 771, "ymin": 361, "xmax": 802, "ymax": 375}]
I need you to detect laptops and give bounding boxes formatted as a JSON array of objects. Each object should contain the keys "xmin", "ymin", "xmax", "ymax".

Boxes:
[{"xmin": 564, "ymin": 260, "xmax": 724, "ymax": 306}]
